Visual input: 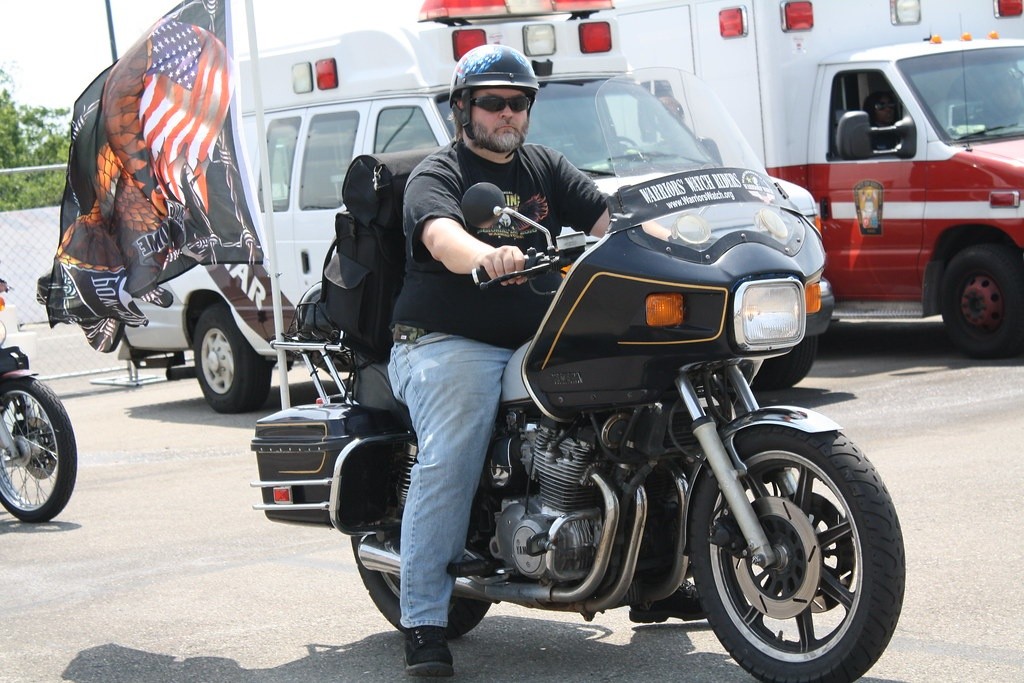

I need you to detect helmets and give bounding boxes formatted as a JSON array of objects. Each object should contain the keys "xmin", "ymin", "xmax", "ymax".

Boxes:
[{"xmin": 449, "ymin": 44, "xmax": 538, "ymax": 126}]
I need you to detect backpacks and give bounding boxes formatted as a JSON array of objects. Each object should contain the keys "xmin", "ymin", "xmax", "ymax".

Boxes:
[{"xmin": 320, "ymin": 143, "xmax": 561, "ymax": 350}]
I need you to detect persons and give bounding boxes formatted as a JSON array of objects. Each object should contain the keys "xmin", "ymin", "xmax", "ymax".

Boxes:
[
  {"xmin": 387, "ymin": 45, "xmax": 609, "ymax": 677},
  {"xmin": 862, "ymin": 91, "xmax": 900, "ymax": 150},
  {"xmin": 650, "ymin": 97, "xmax": 723, "ymax": 166}
]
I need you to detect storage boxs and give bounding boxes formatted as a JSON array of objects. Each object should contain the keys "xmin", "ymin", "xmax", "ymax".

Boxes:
[{"xmin": 250, "ymin": 404, "xmax": 408, "ymax": 529}]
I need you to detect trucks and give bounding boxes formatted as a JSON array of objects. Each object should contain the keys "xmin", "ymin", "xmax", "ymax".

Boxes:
[{"xmin": 409, "ymin": 2, "xmax": 1024, "ymax": 358}]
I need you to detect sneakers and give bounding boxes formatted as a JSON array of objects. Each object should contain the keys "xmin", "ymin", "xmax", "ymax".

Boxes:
[
  {"xmin": 629, "ymin": 579, "xmax": 707, "ymax": 624},
  {"xmin": 405, "ymin": 625, "xmax": 455, "ymax": 678}
]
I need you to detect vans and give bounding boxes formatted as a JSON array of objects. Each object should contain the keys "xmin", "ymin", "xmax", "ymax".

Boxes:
[{"xmin": 111, "ymin": 2, "xmax": 837, "ymax": 418}]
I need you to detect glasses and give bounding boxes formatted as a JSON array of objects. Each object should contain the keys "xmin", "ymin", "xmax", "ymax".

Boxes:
[
  {"xmin": 469, "ymin": 95, "xmax": 531, "ymax": 112},
  {"xmin": 874, "ymin": 99, "xmax": 896, "ymax": 112}
]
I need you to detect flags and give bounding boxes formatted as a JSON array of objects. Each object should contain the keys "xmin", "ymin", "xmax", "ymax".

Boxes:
[{"xmin": 46, "ymin": 0, "xmax": 265, "ymax": 353}]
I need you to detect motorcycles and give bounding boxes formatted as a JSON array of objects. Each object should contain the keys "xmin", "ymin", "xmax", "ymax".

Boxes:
[
  {"xmin": 248, "ymin": 66, "xmax": 907, "ymax": 683},
  {"xmin": 0, "ymin": 275, "xmax": 79, "ymax": 524}
]
